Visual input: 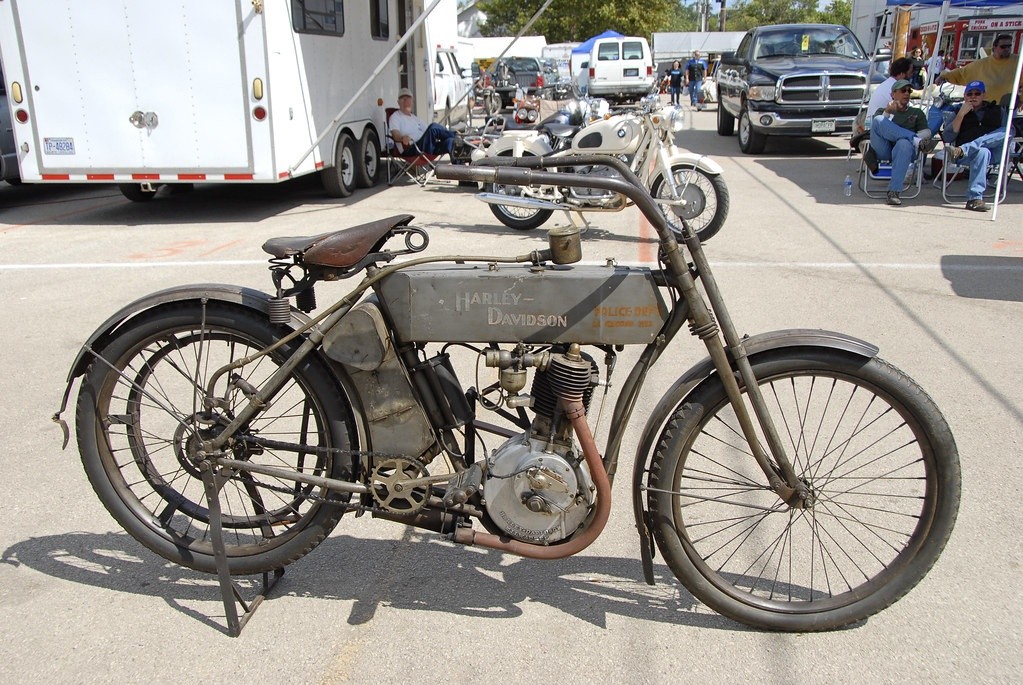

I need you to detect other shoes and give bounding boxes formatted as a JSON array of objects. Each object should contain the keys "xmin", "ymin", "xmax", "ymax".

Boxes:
[{"xmin": 459, "ymin": 179, "xmax": 478, "ymax": 187}]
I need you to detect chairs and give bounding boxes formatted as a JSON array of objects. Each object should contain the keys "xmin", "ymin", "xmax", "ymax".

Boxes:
[
  {"xmin": 855, "ymin": 107, "xmax": 1023, "ymax": 206},
  {"xmin": 385, "ymin": 108, "xmax": 446, "ymax": 186}
]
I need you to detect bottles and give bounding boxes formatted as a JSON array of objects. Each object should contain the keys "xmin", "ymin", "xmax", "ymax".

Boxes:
[{"xmin": 843, "ymin": 175, "xmax": 852, "ymax": 196}]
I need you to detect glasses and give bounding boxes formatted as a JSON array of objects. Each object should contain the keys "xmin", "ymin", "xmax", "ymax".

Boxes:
[
  {"xmin": 996, "ymin": 44, "xmax": 1014, "ymax": 49},
  {"xmin": 896, "ymin": 89, "xmax": 913, "ymax": 94},
  {"xmin": 966, "ymin": 90, "xmax": 982, "ymax": 97}
]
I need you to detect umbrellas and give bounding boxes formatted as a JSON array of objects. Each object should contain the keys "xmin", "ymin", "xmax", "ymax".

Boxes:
[{"xmin": 571, "ymin": 30, "xmax": 628, "ymax": 85}]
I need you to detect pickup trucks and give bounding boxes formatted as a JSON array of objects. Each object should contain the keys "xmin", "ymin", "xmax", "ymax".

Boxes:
[{"xmin": 716, "ymin": 23, "xmax": 892, "ymax": 154}]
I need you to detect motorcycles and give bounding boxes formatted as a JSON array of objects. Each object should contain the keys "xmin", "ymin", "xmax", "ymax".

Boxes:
[
  {"xmin": 51, "ymin": 163, "xmax": 962, "ymax": 634},
  {"xmin": 479, "ymin": 72, "xmax": 502, "ymax": 119},
  {"xmin": 451, "ymin": 80, "xmax": 611, "ymax": 185},
  {"xmin": 512, "ymin": 86, "xmax": 554, "ymax": 125},
  {"xmin": 922, "ymin": 79, "xmax": 1023, "ymax": 167},
  {"xmin": 610, "ymin": 79, "xmax": 667, "ymax": 149},
  {"xmin": 470, "ymin": 94, "xmax": 730, "ymax": 243}
]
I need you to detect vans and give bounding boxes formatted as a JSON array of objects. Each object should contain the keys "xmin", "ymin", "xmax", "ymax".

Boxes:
[{"xmin": 471, "ymin": 55, "xmax": 544, "ymax": 109}]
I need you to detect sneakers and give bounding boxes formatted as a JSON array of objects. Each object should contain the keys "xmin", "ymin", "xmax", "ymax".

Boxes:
[
  {"xmin": 949, "ymin": 146, "xmax": 960, "ymax": 160},
  {"xmin": 919, "ymin": 139, "xmax": 937, "ymax": 154},
  {"xmin": 966, "ymin": 198, "xmax": 990, "ymax": 211},
  {"xmin": 888, "ymin": 190, "xmax": 902, "ymax": 205}
]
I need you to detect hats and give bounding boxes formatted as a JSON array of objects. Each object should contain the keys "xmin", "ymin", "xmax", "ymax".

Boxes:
[
  {"xmin": 965, "ymin": 81, "xmax": 985, "ymax": 92},
  {"xmin": 892, "ymin": 79, "xmax": 914, "ymax": 91},
  {"xmin": 398, "ymin": 88, "xmax": 412, "ymax": 99}
]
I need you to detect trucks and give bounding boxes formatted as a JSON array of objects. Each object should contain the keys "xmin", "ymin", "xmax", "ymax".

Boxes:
[
  {"xmin": 0, "ymin": 1, "xmax": 466, "ymax": 205},
  {"xmin": 588, "ymin": 36, "xmax": 654, "ymax": 100}
]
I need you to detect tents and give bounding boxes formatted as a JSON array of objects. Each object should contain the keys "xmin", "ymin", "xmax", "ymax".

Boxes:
[{"xmin": 846, "ymin": 0, "xmax": 1023, "ymax": 221}]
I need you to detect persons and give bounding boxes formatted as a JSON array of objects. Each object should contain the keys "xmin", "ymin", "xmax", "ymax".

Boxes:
[
  {"xmin": 389, "ymin": 88, "xmax": 477, "ymax": 188},
  {"xmin": 684, "ymin": 50, "xmax": 708, "ymax": 107},
  {"xmin": 865, "ymin": 34, "xmax": 1023, "ymax": 212},
  {"xmin": 660, "ymin": 60, "xmax": 686, "ymax": 105}
]
